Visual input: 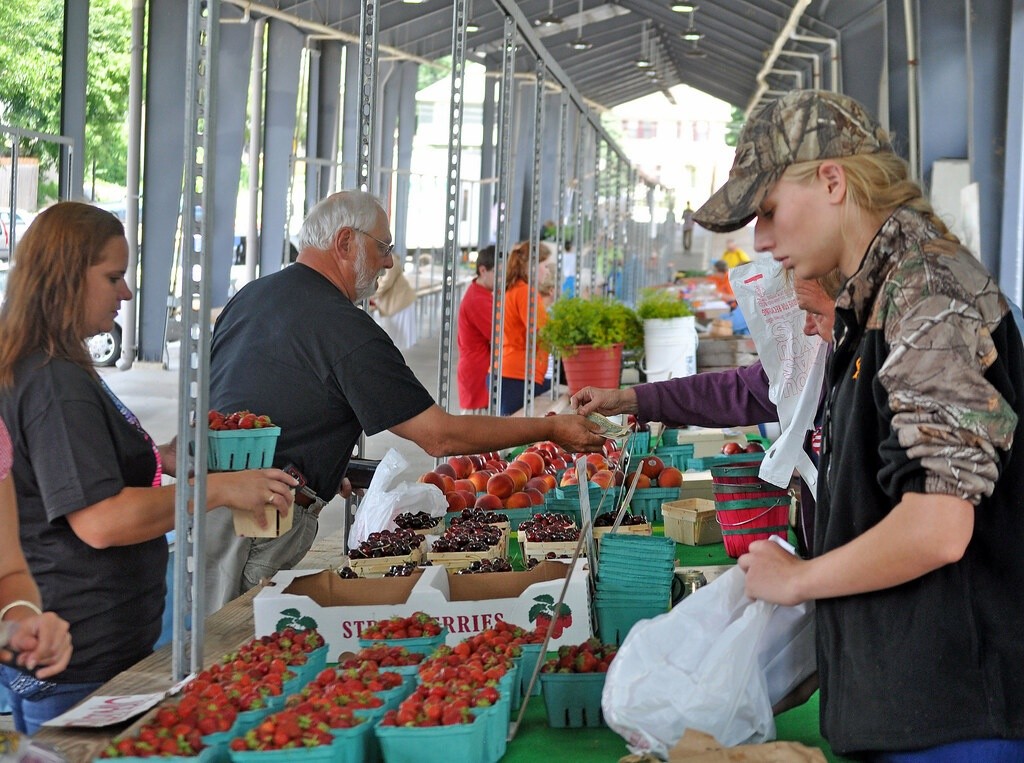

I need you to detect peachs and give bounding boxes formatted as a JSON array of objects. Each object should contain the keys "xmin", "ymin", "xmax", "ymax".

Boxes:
[
  {"xmin": 417, "ymin": 439, "xmax": 625, "ymax": 513},
  {"xmin": 626, "ymin": 456, "xmax": 683, "ymax": 488}
]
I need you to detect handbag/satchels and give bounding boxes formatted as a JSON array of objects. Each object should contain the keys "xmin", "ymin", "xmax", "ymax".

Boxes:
[
  {"xmin": 601, "ymin": 534, "xmax": 818, "ymax": 760},
  {"xmin": 728, "ymin": 258, "xmax": 828, "ymax": 489}
]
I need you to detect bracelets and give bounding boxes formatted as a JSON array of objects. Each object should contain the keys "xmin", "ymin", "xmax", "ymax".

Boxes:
[{"xmin": 0, "ymin": 600, "xmax": 43, "ymax": 619}]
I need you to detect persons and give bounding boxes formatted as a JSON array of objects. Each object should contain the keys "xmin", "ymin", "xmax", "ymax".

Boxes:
[
  {"xmin": 570, "ymin": 89, "xmax": 1024, "ymax": 763},
  {"xmin": 0, "ymin": 203, "xmax": 300, "ymax": 736},
  {"xmin": 202, "ymin": 190, "xmax": 605, "ymax": 616},
  {"xmin": 682, "ymin": 201, "xmax": 695, "ymax": 250}
]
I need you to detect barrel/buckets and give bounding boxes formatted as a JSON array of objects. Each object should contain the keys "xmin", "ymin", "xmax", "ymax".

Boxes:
[
  {"xmin": 711, "ymin": 463, "xmax": 792, "ymax": 558},
  {"xmin": 640, "ymin": 316, "xmax": 699, "ymax": 383}
]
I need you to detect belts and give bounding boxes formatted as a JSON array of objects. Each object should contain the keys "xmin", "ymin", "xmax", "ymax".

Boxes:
[{"xmin": 295, "ymin": 491, "xmax": 324, "ymax": 518}]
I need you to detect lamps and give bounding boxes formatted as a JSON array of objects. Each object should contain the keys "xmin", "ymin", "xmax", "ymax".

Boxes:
[
  {"xmin": 567, "ymin": 0, "xmax": 593, "ymax": 50},
  {"xmin": 671, "ymin": 1, "xmax": 698, "ymax": 12},
  {"xmin": 679, "ymin": 11, "xmax": 705, "ymax": 41},
  {"xmin": 534, "ymin": 0, "xmax": 565, "ymax": 26},
  {"xmin": 636, "ymin": 20, "xmax": 677, "ymax": 104},
  {"xmin": 498, "ymin": 39, "xmax": 522, "ymax": 53},
  {"xmin": 458, "ymin": 0, "xmax": 483, "ymax": 33},
  {"xmin": 684, "ymin": 39, "xmax": 705, "ymax": 58}
]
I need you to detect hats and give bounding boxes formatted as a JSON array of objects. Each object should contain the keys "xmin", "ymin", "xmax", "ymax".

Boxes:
[{"xmin": 691, "ymin": 89, "xmax": 897, "ymax": 234}]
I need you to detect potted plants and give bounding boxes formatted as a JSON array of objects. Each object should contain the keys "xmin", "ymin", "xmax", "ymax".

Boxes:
[
  {"xmin": 638, "ymin": 296, "xmax": 699, "ymax": 385},
  {"xmin": 534, "ymin": 296, "xmax": 643, "ymax": 396}
]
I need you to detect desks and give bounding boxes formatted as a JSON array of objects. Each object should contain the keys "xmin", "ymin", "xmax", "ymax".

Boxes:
[
  {"xmin": 667, "ymin": 283, "xmax": 731, "ymax": 319},
  {"xmin": 30, "ymin": 385, "xmax": 853, "ymax": 763}
]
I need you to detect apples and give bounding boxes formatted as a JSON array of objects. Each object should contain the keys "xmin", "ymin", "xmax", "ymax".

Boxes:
[{"xmin": 721, "ymin": 442, "xmax": 763, "ymax": 454}]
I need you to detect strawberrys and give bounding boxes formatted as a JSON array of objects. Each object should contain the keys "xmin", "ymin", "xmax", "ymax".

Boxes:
[
  {"xmin": 230, "ymin": 641, "xmax": 427, "ymax": 750},
  {"xmin": 361, "ymin": 611, "xmax": 442, "ymax": 639},
  {"xmin": 540, "ymin": 637, "xmax": 619, "ymax": 673},
  {"xmin": 383, "ymin": 620, "xmax": 544, "ymax": 727},
  {"xmin": 98, "ymin": 626, "xmax": 324, "ymax": 757},
  {"xmin": 208, "ymin": 410, "xmax": 272, "ymax": 430}
]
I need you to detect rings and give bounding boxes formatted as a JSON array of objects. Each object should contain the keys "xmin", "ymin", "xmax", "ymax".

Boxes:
[{"xmin": 269, "ymin": 493, "xmax": 275, "ymax": 501}]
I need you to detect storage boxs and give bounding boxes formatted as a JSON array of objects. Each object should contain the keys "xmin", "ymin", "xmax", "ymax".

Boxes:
[
  {"xmin": 696, "ymin": 332, "xmax": 759, "ymax": 374},
  {"xmin": 93, "ymin": 423, "xmax": 794, "ymax": 762}
]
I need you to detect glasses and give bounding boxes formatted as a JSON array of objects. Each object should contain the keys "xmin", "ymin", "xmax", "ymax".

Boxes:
[{"xmin": 355, "ymin": 228, "xmax": 394, "ymax": 256}]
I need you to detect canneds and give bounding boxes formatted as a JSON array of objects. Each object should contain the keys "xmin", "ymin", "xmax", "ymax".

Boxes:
[{"xmin": 670, "ymin": 570, "xmax": 708, "ymax": 609}]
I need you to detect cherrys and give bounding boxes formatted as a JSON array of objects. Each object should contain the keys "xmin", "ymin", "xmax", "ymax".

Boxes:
[{"xmin": 338, "ymin": 511, "xmax": 582, "ymax": 579}]
